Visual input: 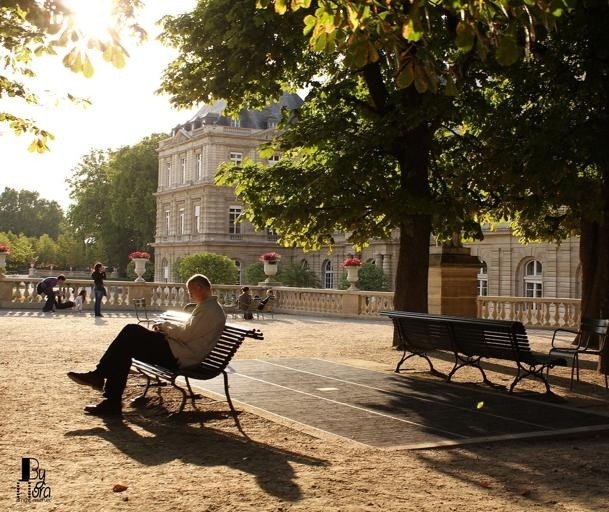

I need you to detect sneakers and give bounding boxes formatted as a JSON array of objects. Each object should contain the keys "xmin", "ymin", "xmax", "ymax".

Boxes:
[
  {"xmin": 66, "ymin": 371, "xmax": 104, "ymax": 391},
  {"xmin": 94, "ymin": 312, "xmax": 104, "ymax": 317},
  {"xmin": 84, "ymin": 400, "xmax": 122, "ymax": 415},
  {"xmin": 42, "ymin": 307, "xmax": 56, "ymax": 312}
]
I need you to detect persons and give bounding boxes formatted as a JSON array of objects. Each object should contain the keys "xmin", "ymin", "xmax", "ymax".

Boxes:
[
  {"xmin": 37, "ymin": 275, "xmax": 66, "ymax": 312},
  {"xmin": 91, "ymin": 263, "xmax": 107, "ymax": 317},
  {"xmin": 255, "ymin": 289, "xmax": 275, "ymax": 310},
  {"xmin": 53, "ymin": 290, "xmax": 87, "ymax": 313},
  {"xmin": 238, "ymin": 286, "xmax": 253, "ymax": 320},
  {"xmin": 67, "ymin": 274, "xmax": 227, "ymax": 416}
]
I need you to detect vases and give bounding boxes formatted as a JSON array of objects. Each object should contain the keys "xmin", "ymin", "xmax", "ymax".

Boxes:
[
  {"xmin": 345, "ymin": 266, "xmax": 360, "ymax": 290},
  {"xmin": 0, "ymin": 251, "xmax": 9, "ymax": 268},
  {"xmin": 133, "ymin": 258, "xmax": 147, "ymax": 282},
  {"xmin": 264, "ymin": 260, "xmax": 278, "ymax": 282}
]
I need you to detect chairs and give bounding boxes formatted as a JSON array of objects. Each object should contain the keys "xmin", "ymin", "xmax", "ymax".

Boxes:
[
  {"xmin": 134, "ymin": 298, "xmax": 157, "ymax": 330},
  {"xmin": 547, "ymin": 318, "xmax": 609, "ymax": 392}
]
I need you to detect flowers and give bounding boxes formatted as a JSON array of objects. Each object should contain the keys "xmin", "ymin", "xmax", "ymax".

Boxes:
[
  {"xmin": 129, "ymin": 252, "xmax": 150, "ymax": 258},
  {"xmin": 0, "ymin": 242, "xmax": 10, "ymax": 252},
  {"xmin": 258, "ymin": 251, "xmax": 282, "ymax": 260},
  {"xmin": 342, "ymin": 258, "xmax": 362, "ymax": 266}
]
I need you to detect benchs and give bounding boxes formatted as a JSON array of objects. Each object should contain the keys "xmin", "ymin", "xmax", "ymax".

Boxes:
[
  {"xmin": 132, "ymin": 312, "xmax": 264, "ymax": 425},
  {"xmin": 222, "ymin": 298, "xmax": 277, "ymax": 319},
  {"xmin": 379, "ymin": 310, "xmax": 569, "ymax": 403}
]
